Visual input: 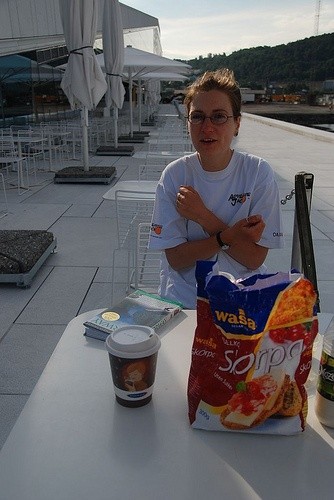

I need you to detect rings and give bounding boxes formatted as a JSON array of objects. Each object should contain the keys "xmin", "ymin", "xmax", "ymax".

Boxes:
[{"xmin": 246, "ymin": 217, "xmax": 249, "ymax": 223}]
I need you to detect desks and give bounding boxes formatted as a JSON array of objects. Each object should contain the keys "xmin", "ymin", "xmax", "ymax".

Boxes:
[
  {"xmin": 134, "ymin": 151, "xmax": 192, "ymax": 158},
  {"xmin": 0, "ymin": 310, "xmax": 334, "ymax": 500},
  {"xmin": 102, "ymin": 180, "xmax": 159, "ymax": 200},
  {"xmin": 149, "ymin": 139, "xmax": 192, "ymax": 145},
  {"xmin": 0, "ymin": 136, "xmax": 48, "ymax": 142},
  {"xmin": 0, "ymin": 129, "xmax": 71, "ymax": 136}
]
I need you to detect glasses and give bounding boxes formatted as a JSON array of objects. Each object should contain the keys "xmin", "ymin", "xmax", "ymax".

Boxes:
[{"xmin": 184, "ymin": 111, "xmax": 235, "ymax": 125}]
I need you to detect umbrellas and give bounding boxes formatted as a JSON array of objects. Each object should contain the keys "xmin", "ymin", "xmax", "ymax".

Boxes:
[
  {"xmin": 55, "ymin": 44, "xmax": 192, "ymax": 138},
  {"xmin": 99, "ymin": 0, "xmax": 126, "ymax": 148},
  {"xmin": 59, "ymin": 0, "xmax": 108, "ymax": 172},
  {"xmin": 0, "ymin": 54, "xmax": 64, "ymax": 82}
]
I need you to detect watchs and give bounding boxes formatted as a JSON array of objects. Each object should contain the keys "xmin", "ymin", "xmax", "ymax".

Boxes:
[{"xmin": 216, "ymin": 231, "xmax": 230, "ymax": 250}]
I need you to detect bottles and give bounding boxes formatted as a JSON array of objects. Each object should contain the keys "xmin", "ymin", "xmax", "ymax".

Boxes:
[{"xmin": 315, "ymin": 316, "xmax": 334, "ymax": 430}]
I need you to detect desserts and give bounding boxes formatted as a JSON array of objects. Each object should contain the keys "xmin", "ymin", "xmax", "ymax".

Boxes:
[{"xmin": 221, "ymin": 366, "xmax": 303, "ymax": 430}]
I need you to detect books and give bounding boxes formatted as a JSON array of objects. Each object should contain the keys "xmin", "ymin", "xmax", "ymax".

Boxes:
[{"xmin": 83, "ymin": 289, "xmax": 183, "ymax": 342}]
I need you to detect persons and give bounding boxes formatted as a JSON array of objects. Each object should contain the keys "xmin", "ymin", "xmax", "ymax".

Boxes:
[{"xmin": 147, "ymin": 68, "xmax": 281, "ymax": 310}]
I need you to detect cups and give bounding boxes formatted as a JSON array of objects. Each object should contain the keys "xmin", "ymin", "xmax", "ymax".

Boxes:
[{"xmin": 106, "ymin": 325, "xmax": 162, "ymax": 408}]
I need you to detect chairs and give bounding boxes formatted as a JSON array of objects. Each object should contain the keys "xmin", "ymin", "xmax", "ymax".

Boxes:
[
  {"xmin": 110, "ymin": 115, "xmax": 196, "ymax": 308},
  {"xmin": 0, "ymin": 117, "xmax": 120, "ymax": 216}
]
